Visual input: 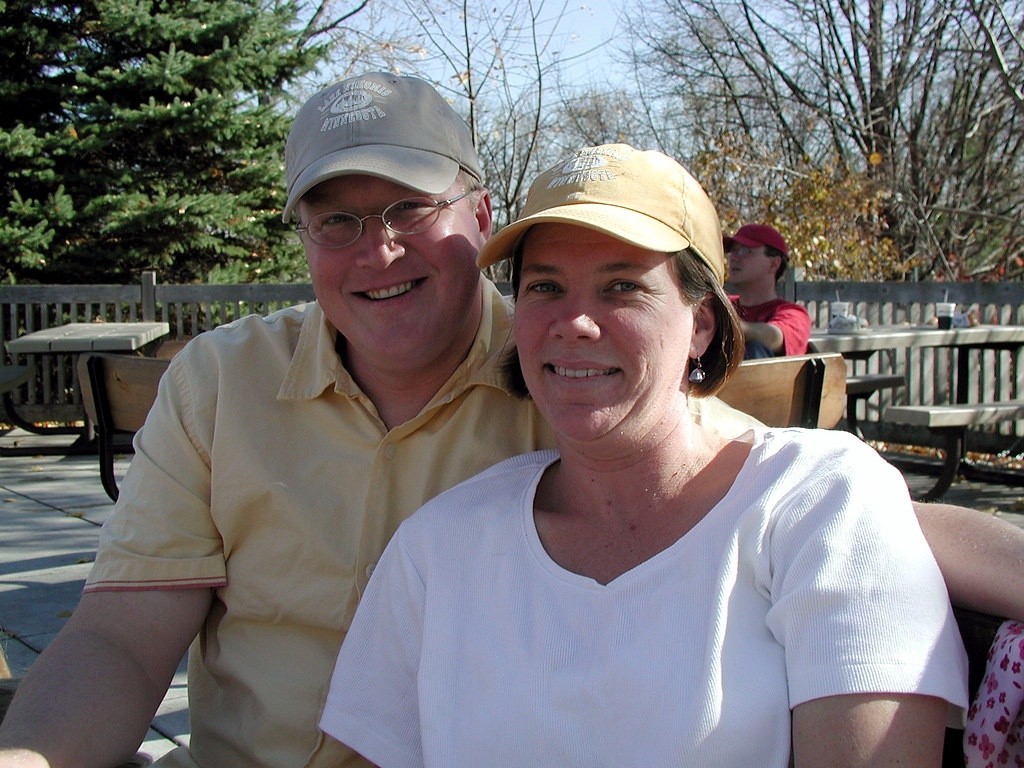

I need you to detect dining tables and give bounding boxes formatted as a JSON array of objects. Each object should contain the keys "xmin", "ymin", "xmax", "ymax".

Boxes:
[
  {"xmin": 4, "ymin": 321, "xmax": 171, "ymax": 355},
  {"xmin": 795, "ymin": 322, "xmax": 1024, "ymax": 483}
]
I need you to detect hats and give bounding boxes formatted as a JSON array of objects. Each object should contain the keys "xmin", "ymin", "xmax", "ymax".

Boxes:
[
  {"xmin": 282, "ymin": 73, "xmax": 481, "ymax": 223},
  {"xmin": 477, "ymin": 144, "xmax": 726, "ymax": 290},
  {"xmin": 722, "ymin": 223, "xmax": 790, "ymax": 261}
]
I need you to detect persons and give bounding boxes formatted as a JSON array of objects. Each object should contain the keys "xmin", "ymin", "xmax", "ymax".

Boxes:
[
  {"xmin": 718, "ymin": 223, "xmax": 811, "ymax": 363},
  {"xmin": 0, "ymin": 70, "xmax": 1024, "ymax": 768},
  {"xmin": 301, "ymin": 139, "xmax": 972, "ymax": 767}
]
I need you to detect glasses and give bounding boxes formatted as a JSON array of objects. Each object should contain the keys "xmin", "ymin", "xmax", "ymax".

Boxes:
[{"xmin": 295, "ymin": 185, "xmax": 478, "ymax": 248}]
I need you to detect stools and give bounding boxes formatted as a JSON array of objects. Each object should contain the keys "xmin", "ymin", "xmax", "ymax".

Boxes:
[
  {"xmin": 885, "ymin": 397, "xmax": 1024, "ymax": 502},
  {"xmin": 843, "ymin": 373, "xmax": 906, "ymax": 444}
]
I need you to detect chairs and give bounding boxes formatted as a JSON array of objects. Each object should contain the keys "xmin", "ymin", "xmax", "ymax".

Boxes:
[
  {"xmin": 695, "ymin": 353, "xmax": 849, "ymax": 429},
  {"xmin": 0, "ymin": 337, "xmax": 207, "ymax": 504}
]
